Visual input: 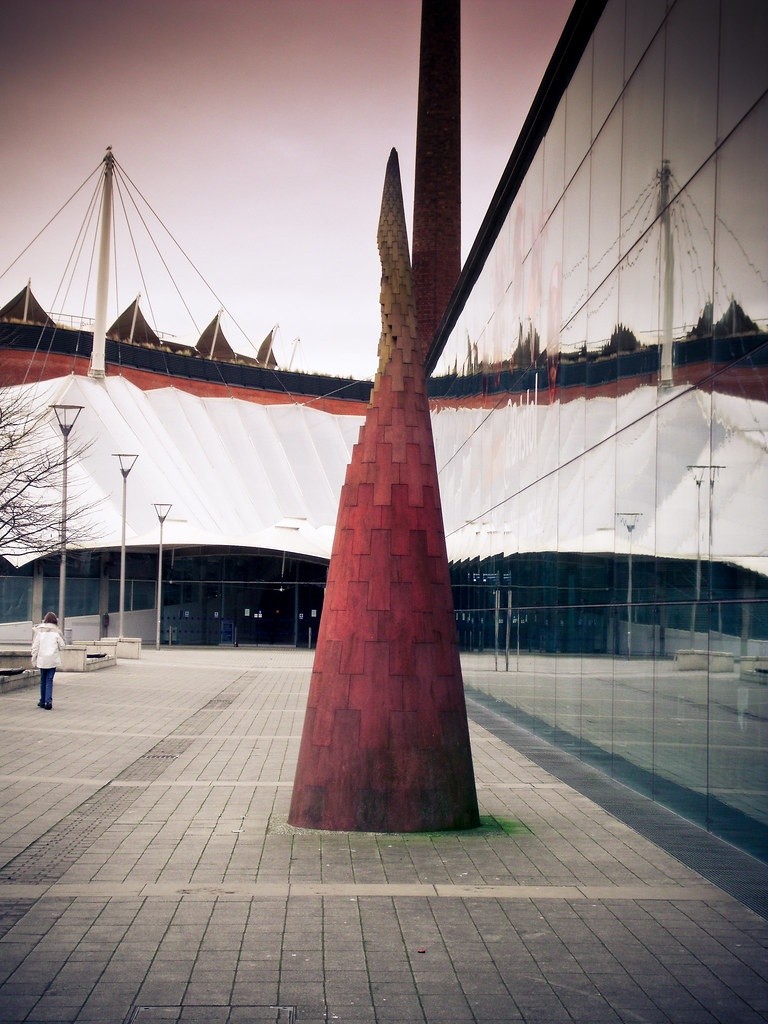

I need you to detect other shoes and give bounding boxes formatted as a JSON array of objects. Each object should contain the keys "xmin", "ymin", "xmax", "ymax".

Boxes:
[
  {"xmin": 45, "ymin": 701, "xmax": 52, "ymax": 710},
  {"xmin": 38, "ymin": 701, "xmax": 45, "ymax": 708}
]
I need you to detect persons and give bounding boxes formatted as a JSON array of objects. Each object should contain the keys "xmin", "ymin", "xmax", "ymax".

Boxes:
[
  {"xmin": 31, "ymin": 611, "xmax": 65, "ymax": 710},
  {"xmin": 102, "ymin": 612, "xmax": 109, "ymax": 637}
]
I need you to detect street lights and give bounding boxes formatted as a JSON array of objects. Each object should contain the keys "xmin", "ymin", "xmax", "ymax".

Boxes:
[
  {"xmin": 109, "ymin": 453, "xmax": 140, "ymax": 640},
  {"xmin": 614, "ymin": 511, "xmax": 645, "ymax": 659},
  {"xmin": 152, "ymin": 503, "xmax": 173, "ymax": 651},
  {"xmin": 685, "ymin": 463, "xmax": 724, "ymax": 632},
  {"xmin": 48, "ymin": 404, "xmax": 87, "ymax": 636}
]
